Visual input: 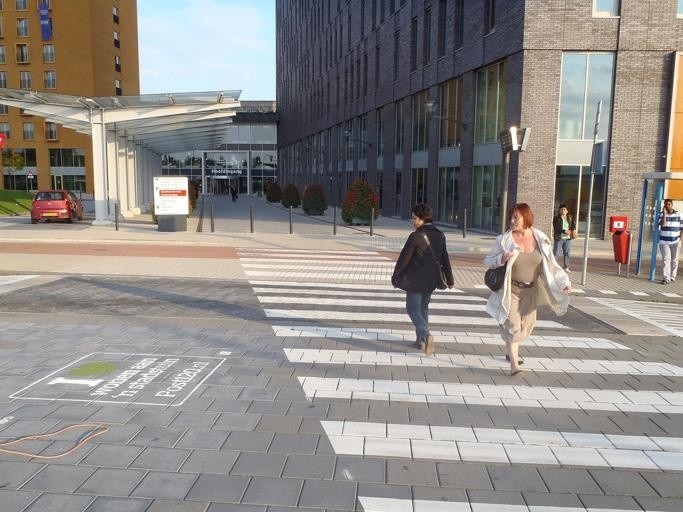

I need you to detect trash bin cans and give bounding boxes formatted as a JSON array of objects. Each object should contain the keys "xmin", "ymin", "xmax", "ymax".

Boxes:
[{"xmin": 612, "ymin": 229, "xmax": 630, "ymax": 264}]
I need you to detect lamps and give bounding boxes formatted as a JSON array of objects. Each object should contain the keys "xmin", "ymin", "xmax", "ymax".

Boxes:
[
  {"xmin": 267, "ymin": 127, "xmax": 374, "ymax": 162},
  {"xmin": 424, "ymin": 97, "xmax": 470, "ymax": 129}
]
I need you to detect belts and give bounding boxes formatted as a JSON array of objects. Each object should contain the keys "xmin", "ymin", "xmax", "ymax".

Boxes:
[{"xmin": 512, "ymin": 280, "xmax": 537, "ymax": 289}]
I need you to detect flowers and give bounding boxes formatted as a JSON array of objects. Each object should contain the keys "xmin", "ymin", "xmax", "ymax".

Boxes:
[
  {"xmin": 338, "ymin": 175, "xmax": 382, "ymax": 224},
  {"xmin": 265, "ymin": 181, "xmax": 329, "ymax": 217}
]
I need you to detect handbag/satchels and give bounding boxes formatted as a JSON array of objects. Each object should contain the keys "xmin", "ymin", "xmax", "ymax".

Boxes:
[
  {"xmin": 570, "ymin": 228, "xmax": 577, "ymax": 239},
  {"xmin": 484, "ymin": 266, "xmax": 506, "ymax": 292},
  {"xmin": 434, "ymin": 261, "xmax": 447, "ymax": 289}
]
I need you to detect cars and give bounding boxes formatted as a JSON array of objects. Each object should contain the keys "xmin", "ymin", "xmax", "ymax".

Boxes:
[{"xmin": 31, "ymin": 192, "xmax": 84, "ymax": 225}]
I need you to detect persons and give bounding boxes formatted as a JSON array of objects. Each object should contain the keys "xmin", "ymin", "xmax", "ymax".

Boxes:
[
  {"xmin": 391, "ymin": 204, "xmax": 453, "ymax": 355},
  {"xmin": 230, "ymin": 185, "xmax": 237, "ymax": 202},
  {"xmin": 552, "ymin": 203, "xmax": 577, "ymax": 274},
  {"xmin": 482, "ymin": 203, "xmax": 572, "ymax": 375},
  {"xmin": 656, "ymin": 199, "xmax": 683, "ymax": 283}
]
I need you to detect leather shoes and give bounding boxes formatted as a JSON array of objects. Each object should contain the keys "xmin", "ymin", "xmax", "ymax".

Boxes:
[{"xmin": 506, "ymin": 352, "xmax": 524, "ymax": 376}]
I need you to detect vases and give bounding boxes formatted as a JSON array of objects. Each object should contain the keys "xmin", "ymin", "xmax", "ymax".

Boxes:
[{"xmin": 348, "ymin": 216, "xmax": 371, "ymax": 227}]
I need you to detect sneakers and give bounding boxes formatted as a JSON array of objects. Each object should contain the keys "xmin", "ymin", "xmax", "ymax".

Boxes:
[
  {"xmin": 660, "ymin": 276, "xmax": 676, "ymax": 284},
  {"xmin": 416, "ymin": 334, "xmax": 434, "ymax": 355},
  {"xmin": 564, "ymin": 268, "xmax": 571, "ymax": 273}
]
